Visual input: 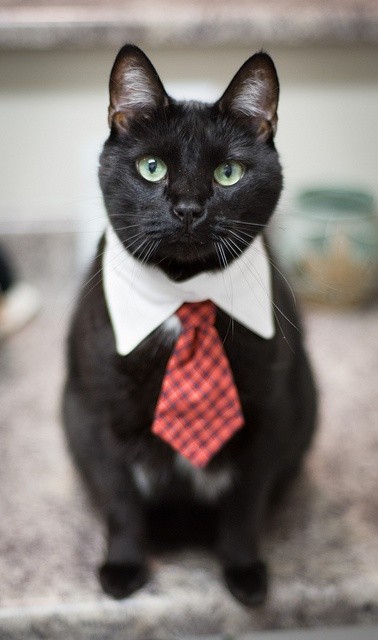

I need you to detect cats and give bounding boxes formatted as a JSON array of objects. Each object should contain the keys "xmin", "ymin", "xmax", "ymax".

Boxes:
[{"xmin": 61, "ymin": 42, "xmax": 315, "ymax": 608}]
